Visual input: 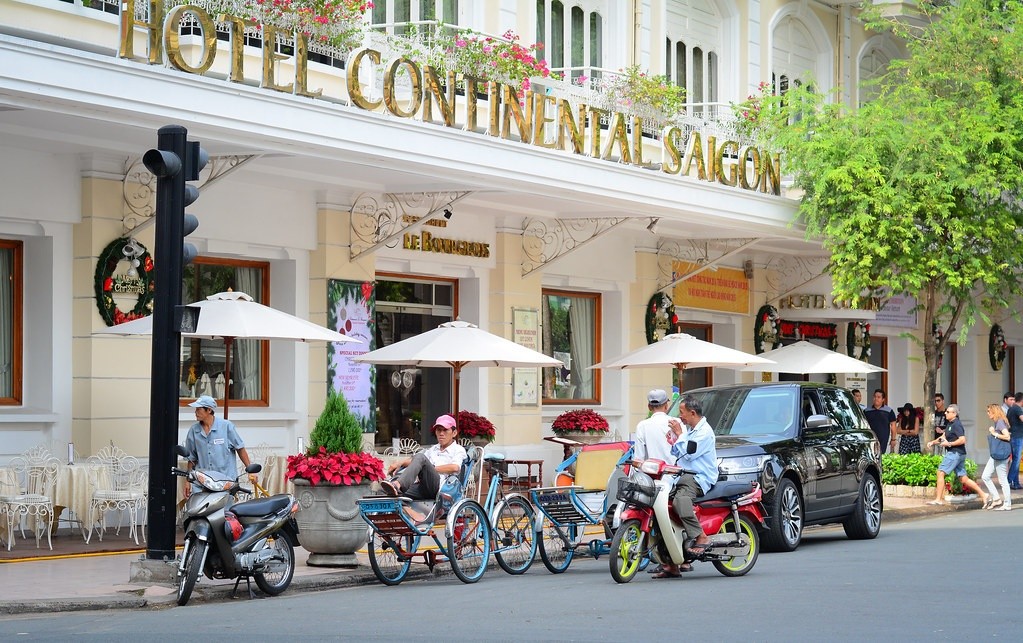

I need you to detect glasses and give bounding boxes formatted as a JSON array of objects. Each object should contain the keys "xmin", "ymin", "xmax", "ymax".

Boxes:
[
  {"xmin": 945, "ymin": 410, "xmax": 954, "ymax": 414},
  {"xmin": 935, "ymin": 400, "xmax": 940, "ymax": 403}
]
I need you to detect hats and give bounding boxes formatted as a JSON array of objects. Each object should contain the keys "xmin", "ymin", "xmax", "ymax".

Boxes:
[
  {"xmin": 188, "ymin": 395, "xmax": 218, "ymax": 411},
  {"xmin": 647, "ymin": 389, "xmax": 670, "ymax": 406},
  {"xmin": 897, "ymin": 403, "xmax": 916, "ymax": 417},
  {"xmin": 433, "ymin": 415, "xmax": 456, "ymax": 429}
]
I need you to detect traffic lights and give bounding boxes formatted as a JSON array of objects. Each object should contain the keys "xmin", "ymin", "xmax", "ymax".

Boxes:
[
  {"xmin": 142, "ymin": 148, "xmax": 183, "ymax": 181},
  {"xmin": 186, "ymin": 140, "xmax": 209, "ymax": 182},
  {"xmin": 183, "ymin": 183, "xmax": 199, "ymax": 271}
]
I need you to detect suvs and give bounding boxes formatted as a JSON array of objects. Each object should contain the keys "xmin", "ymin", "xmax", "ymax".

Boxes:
[{"xmin": 666, "ymin": 380, "xmax": 885, "ymax": 554}]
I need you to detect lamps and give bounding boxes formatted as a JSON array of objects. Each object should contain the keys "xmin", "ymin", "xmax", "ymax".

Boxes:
[{"xmin": 645, "ymin": 217, "xmax": 661, "ymax": 234}]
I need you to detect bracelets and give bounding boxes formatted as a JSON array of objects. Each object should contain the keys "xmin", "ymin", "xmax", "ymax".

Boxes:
[
  {"xmin": 892, "ymin": 439, "xmax": 896, "ymax": 442},
  {"xmin": 909, "ymin": 430, "xmax": 910, "ymax": 432},
  {"xmin": 993, "ymin": 433, "xmax": 999, "ymax": 438}
]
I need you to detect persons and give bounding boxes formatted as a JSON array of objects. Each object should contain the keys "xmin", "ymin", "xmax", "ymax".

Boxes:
[
  {"xmin": 635, "ymin": 390, "xmax": 719, "ymax": 579},
  {"xmin": 851, "ymin": 389, "xmax": 1023, "ymax": 511},
  {"xmin": 376, "ymin": 415, "xmax": 467, "ymax": 500},
  {"xmin": 184, "ymin": 395, "xmax": 258, "ymax": 502}
]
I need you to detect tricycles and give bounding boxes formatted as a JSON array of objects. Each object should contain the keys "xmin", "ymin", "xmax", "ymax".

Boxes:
[
  {"xmin": 527, "ymin": 439, "xmax": 636, "ymax": 574},
  {"xmin": 355, "ymin": 442, "xmax": 541, "ymax": 586}
]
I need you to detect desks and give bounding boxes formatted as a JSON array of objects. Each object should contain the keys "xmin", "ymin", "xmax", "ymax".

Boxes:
[
  {"xmin": 175, "ymin": 460, "xmax": 191, "ymax": 525},
  {"xmin": 0, "ymin": 468, "xmax": 22, "ymax": 547},
  {"xmin": 262, "ymin": 455, "xmax": 294, "ymax": 496},
  {"xmin": 28, "ymin": 464, "xmax": 110, "ymax": 544},
  {"xmin": 500, "ymin": 460, "xmax": 544, "ymax": 502}
]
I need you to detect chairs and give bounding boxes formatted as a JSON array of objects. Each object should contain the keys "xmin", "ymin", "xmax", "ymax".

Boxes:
[
  {"xmin": 554, "ymin": 441, "xmax": 634, "ymax": 495},
  {"xmin": 236, "ymin": 441, "xmax": 276, "ymax": 504},
  {"xmin": 412, "ymin": 459, "xmax": 474, "ymax": 503},
  {"xmin": 0, "ymin": 445, "xmax": 148, "ymax": 551}
]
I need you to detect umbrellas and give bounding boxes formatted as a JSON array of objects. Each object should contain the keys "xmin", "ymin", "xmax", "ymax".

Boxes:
[
  {"xmin": 350, "ymin": 316, "xmax": 563, "ymax": 448},
  {"xmin": 90, "ymin": 288, "xmax": 364, "ymax": 420},
  {"xmin": 585, "ymin": 333, "xmax": 778, "ymax": 394},
  {"xmin": 716, "ymin": 341, "xmax": 888, "ymax": 382}
]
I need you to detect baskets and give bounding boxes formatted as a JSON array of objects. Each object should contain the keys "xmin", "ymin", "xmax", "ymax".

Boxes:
[{"xmin": 616, "ymin": 477, "xmax": 661, "ymax": 508}]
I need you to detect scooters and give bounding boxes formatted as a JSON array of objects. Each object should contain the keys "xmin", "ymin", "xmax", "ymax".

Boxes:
[
  {"xmin": 171, "ymin": 445, "xmax": 301, "ymax": 606},
  {"xmin": 608, "ymin": 458, "xmax": 771, "ymax": 583}
]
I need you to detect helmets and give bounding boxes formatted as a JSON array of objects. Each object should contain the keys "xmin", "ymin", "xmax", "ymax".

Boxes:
[
  {"xmin": 225, "ymin": 517, "xmax": 244, "ymax": 541},
  {"xmin": 625, "ymin": 473, "xmax": 656, "ymax": 505}
]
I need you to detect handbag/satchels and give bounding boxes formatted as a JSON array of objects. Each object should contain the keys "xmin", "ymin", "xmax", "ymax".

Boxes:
[{"xmin": 987, "ymin": 420, "xmax": 1012, "ymax": 460}]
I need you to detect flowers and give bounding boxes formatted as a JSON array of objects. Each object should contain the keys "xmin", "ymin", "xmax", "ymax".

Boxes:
[
  {"xmin": 577, "ymin": 64, "xmax": 688, "ymax": 126},
  {"xmin": 552, "ymin": 408, "xmax": 610, "ymax": 436},
  {"xmin": 162, "ymin": 0, "xmax": 374, "ymax": 49},
  {"xmin": 282, "ymin": 388, "xmax": 386, "ymax": 486},
  {"xmin": 429, "ymin": 409, "xmax": 496, "ymax": 444},
  {"xmin": 715, "ymin": 81, "xmax": 783, "ymax": 140},
  {"xmin": 380, "ymin": 17, "xmax": 565, "ymax": 108}
]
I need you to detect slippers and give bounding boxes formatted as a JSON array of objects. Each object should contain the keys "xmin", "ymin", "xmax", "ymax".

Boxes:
[
  {"xmin": 652, "ymin": 570, "xmax": 682, "ymax": 579},
  {"xmin": 647, "ymin": 564, "xmax": 664, "ymax": 573},
  {"xmin": 687, "ymin": 540, "xmax": 714, "ymax": 555},
  {"xmin": 679, "ymin": 562, "xmax": 694, "ymax": 572}
]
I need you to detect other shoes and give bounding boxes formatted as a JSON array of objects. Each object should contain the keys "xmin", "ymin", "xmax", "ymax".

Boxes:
[
  {"xmin": 380, "ymin": 481, "xmax": 399, "ymax": 496},
  {"xmin": 981, "ymin": 492, "xmax": 992, "ymax": 509},
  {"xmin": 987, "ymin": 500, "xmax": 1002, "ymax": 510},
  {"xmin": 1008, "ymin": 480, "xmax": 1023, "ymax": 489},
  {"xmin": 926, "ymin": 500, "xmax": 946, "ymax": 506},
  {"xmin": 994, "ymin": 503, "xmax": 1012, "ymax": 511}
]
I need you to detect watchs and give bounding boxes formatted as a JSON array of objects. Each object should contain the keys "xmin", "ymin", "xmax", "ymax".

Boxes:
[{"xmin": 949, "ymin": 442, "xmax": 951, "ymax": 447}]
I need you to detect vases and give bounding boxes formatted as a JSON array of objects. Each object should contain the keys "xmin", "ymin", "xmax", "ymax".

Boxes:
[
  {"xmin": 458, "ymin": 436, "xmax": 490, "ymax": 482},
  {"xmin": 556, "ymin": 431, "xmax": 604, "ymax": 474},
  {"xmin": 291, "ymin": 479, "xmax": 372, "ymax": 566}
]
